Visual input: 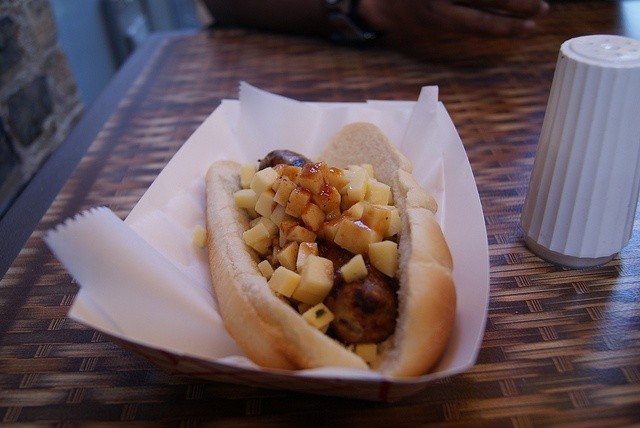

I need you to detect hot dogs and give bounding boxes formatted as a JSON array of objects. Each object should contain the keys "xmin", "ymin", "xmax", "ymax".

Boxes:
[{"xmin": 206, "ymin": 122, "xmax": 456, "ymax": 380}]
